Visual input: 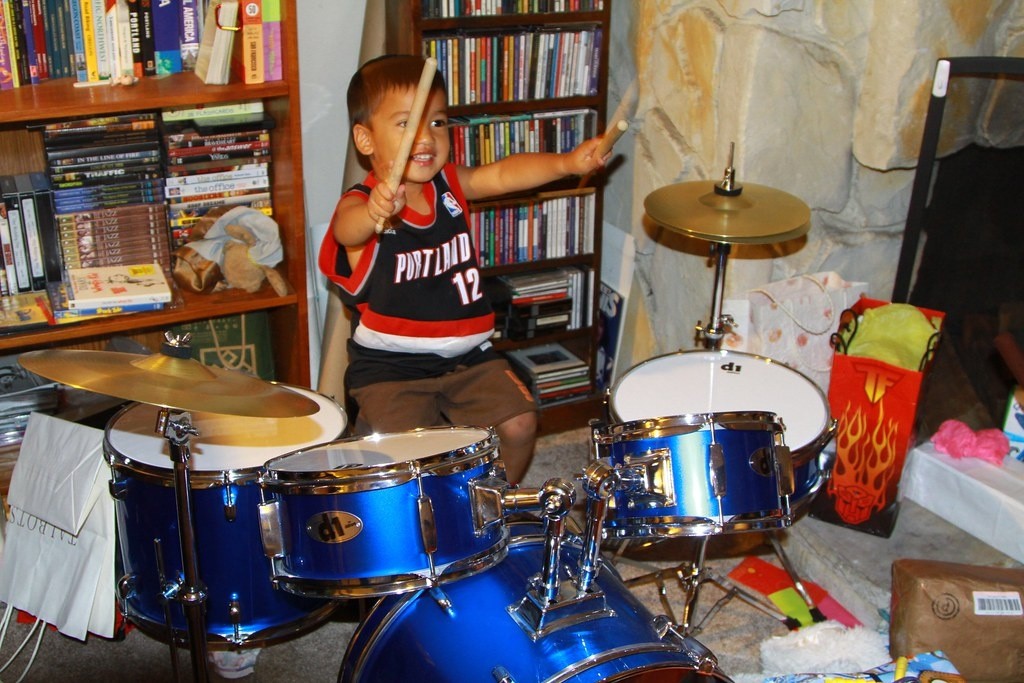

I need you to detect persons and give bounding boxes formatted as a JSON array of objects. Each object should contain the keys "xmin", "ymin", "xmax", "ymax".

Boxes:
[{"xmin": 318, "ymin": 53, "xmax": 613, "ymax": 488}]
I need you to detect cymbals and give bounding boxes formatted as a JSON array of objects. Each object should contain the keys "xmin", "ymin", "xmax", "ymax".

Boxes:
[
  {"xmin": 644, "ymin": 178, "xmax": 811, "ymax": 244},
  {"xmin": 17, "ymin": 347, "xmax": 320, "ymax": 420}
]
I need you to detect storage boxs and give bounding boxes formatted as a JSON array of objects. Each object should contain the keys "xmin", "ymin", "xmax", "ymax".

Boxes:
[{"xmin": 889, "ymin": 559, "xmax": 1024, "ymax": 683}]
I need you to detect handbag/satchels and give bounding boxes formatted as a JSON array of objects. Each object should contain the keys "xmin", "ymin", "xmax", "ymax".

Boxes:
[
  {"xmin": 717, "ymin": 271, "xmax": 1024, "ymax": 539},
  {"xmin": 0, "ymin": 411, "xmax": 115, "ymax": 683}
]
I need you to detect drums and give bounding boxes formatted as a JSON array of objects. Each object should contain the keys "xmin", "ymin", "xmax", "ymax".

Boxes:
[
  {"xmin": 335, "ymin": 522, "xmax": 737, "ymax": 683},
  {"xmin": 260, "ymin": 422, "xmax": 510, "ymax": 599},
  {"xmin": 613, "ymin": 349, "xmax": 833, "ymax": 509},
  {"xmin": 102, "ymin": 383, "xmax": 350, "ymax": 650},
  {"xmin": 581, "ymin": 410, "xmax": 794, "ymax": 540}
]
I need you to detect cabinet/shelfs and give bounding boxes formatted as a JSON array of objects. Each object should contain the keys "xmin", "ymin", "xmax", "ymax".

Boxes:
[
  {"xmin": 386, "ymin": 0, "xmax": 611, "ymax": 435},
  {"xmin": 904, "ymin": 440, "xmax": 1024, "ymax": 565},
  {"xmin": 0, "ymin": 0, "xmax": 312, "ymax": 493}
]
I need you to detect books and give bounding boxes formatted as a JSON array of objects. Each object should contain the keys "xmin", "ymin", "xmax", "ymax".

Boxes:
[
  {"xmin": 421, "ymin": 0, "xmax": 604, "ymax": 410},
  {"xmin": 0, "ymin": 0, "xmax": 283, "ymax": 336}
]
